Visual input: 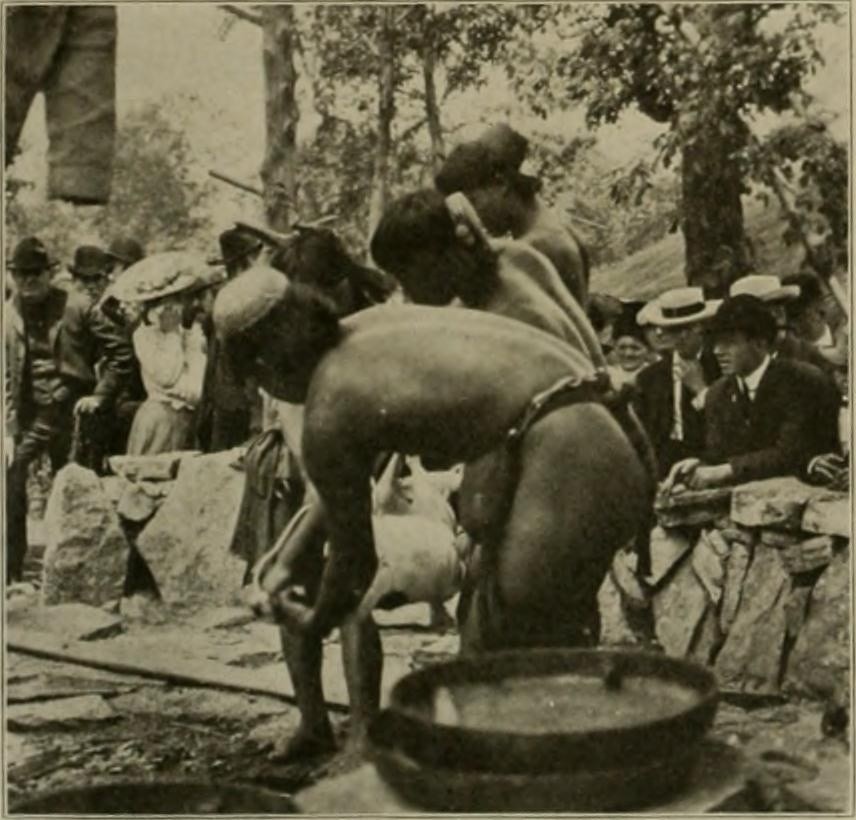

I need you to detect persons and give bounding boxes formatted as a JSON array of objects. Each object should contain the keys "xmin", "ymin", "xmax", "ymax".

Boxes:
[
  {"xmin": 254, "ymin": 225, "xmax": 414, "ymax": 783},
  {"xmin": 6, "ymin": 121, "xmax": 852, "ymax": 632},
  {"xmin": 214, "ymin": 266, "xmax": 660, "ymax": 656}
]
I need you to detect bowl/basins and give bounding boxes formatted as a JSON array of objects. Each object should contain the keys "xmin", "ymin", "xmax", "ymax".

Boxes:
[
  {"xmin": 358, "ymin": 650, "xmax": 718, "ymax": 813},
  {"xmin": 8, "ymin": 783, "xmax": 304, "ymax": 814}
]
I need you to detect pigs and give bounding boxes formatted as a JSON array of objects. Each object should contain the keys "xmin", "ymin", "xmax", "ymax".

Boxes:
[{"xmin": 243, "ymin": 455, "xmax": 467, "ymax": 618}]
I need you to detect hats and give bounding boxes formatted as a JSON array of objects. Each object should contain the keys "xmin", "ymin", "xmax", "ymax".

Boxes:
[
  {"xmin": 729, "ymin": 274, "xmax": 802, "ymax": 308},
  {"xmin": 638, "ymin": 285, "xmax": 722, "ymax": 323},
  {"xmin": 69, "ymin": 245, "xmax": 111, "ymax": 275},
  {"xmin": 6, "ymin": 238, "xmax": 58, "ymax": 270},
  {"xmin": 702, "ymin": 289, "xmax": 788, "ymax": 335},
  {"xmin": 111, "ymin": 254, "xmax": 207, "ymax": 301},
  {"xmin": 204, "ymin": 228, "xmax": 264, "ymax": 265},
  {"xmin": 611, "ymin": 298, "xmax": 648, "ymax": 345},
  {"xmin": 105, "ymin": 238, "xmax": 145, "ymax": 265},
  {"xmin": 214, "ymin": 264, "xmax": 289, "ymax": 336}
]
